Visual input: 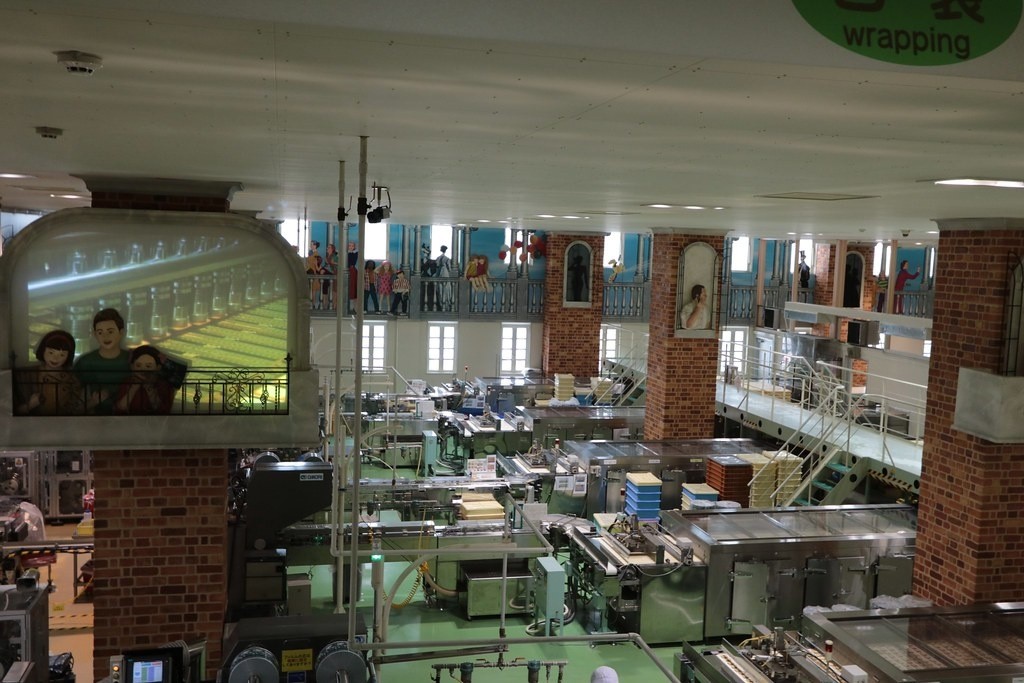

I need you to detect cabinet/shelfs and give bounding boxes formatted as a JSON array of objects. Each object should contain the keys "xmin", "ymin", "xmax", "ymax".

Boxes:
[{"xmin": 0, "ymin": 581, "xmax": 56, "ymax": 683}]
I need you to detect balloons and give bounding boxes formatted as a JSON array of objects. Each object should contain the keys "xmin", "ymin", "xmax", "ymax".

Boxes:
[{"xmin": 499, "ymin": 230, "xmax": 547, "ymax": 263}]
